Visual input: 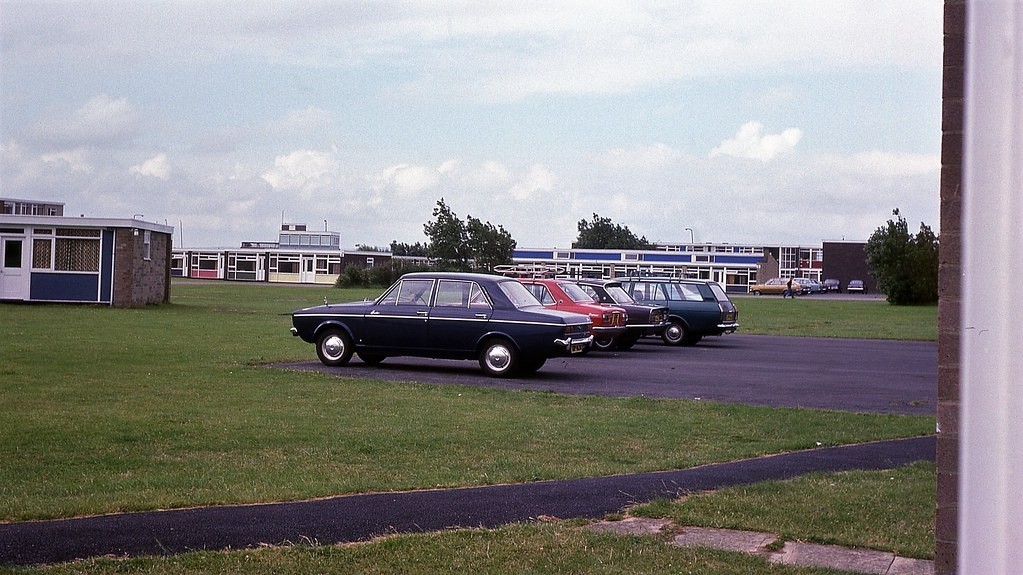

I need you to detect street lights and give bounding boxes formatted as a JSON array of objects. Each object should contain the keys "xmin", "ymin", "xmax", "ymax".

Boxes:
[
  {"xmin": 324, "ymin": 220, "xmax": 327, "ymax": 232},
  {"xmin": 685, "ymin": 228, "xmax": 693, "ymax": 243},
  {"xmin": 134, "ymin": 214, "xmax": 144, "ymax": 220}
]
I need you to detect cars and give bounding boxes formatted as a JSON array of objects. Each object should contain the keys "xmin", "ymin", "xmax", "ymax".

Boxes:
[
  {"xmin": 469, "ymin": 275, "xmax": 628, "ymax": 357},
  {"xmin": 289, "ymin": 269, "xmax": 596, "ymax": 379},
  {"xmin": 822, "ymin": 278, "xmax": 843, "ymax": 294},
  {"xmin": 750, "ymin": 278, "xmax": 803, "ymax": 297},
  {"xmin": 847, "ymin": 279, "xmax": 868, "ymax": 294},
  {"xmin": 793, "ymin": 277, "xmax": 824, "ymax": 295},
  {"xmin": 610, "ymin": 277, "xmax": 740, "ymax": 347},
  {"xmin": 541, "ymin": 277, "xmax": 672, "ymax": 351}
]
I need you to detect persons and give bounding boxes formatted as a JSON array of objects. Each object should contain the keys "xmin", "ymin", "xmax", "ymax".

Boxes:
[{"xmin": 783, "ymin": 276, "xmax": 795, "ymax": 298}]
